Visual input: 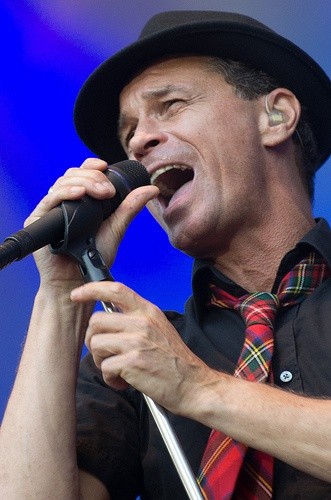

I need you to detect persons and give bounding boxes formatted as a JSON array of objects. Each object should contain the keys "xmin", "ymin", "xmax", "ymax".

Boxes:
[{"xmin": 0, "ymin": 10, "xmax": 331, "ymax": 500}]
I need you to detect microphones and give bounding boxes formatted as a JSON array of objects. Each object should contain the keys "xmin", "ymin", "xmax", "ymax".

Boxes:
[{"xmin": 0, "ymin": 160, "xmax": 151, "ymax": 272}]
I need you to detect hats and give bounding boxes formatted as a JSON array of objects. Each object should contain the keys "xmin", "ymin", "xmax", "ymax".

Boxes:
[{"xmin": 73, "ymin": 9, "xmax": 331, "ymax": 171}]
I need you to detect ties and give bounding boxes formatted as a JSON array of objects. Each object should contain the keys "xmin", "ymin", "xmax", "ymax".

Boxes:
[{"xmin": 197, "ymin": 251, "xmax": 331, "ymax": 500}]
